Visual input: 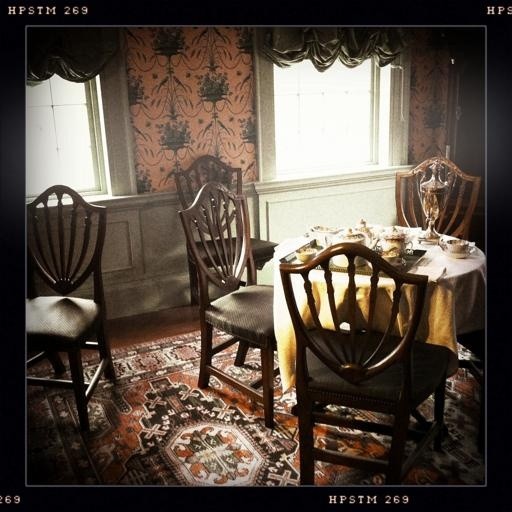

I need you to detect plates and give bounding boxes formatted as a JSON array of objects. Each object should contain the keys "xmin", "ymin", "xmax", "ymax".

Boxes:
[{"xmin": 441, "ymin": 246, "xmax": 476, "ymax": 258}]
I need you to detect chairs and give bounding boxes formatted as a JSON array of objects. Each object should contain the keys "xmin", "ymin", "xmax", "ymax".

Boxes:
[
  {"xmin": 27, "ymin": 185, "xmax": 117, "ymax": 432},
  {"xmin": 172, "ymin": 147, "xmax": 487, "ymax": 487}
]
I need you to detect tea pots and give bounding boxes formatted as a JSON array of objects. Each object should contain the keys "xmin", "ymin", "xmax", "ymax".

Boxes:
[{"xmin": 324, "ymin": 230, "xmax": 380, "ymax": 267}]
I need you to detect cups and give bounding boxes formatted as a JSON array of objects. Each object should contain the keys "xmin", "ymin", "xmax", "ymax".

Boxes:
[
  {"xmin": 380, "ymin": 234, "xmax": 413, "ymax": 268},
  {"xmin": 448, "ymin": 240, "xmax": 469, "ymax": 252},
  {"xmin": 296, "ymin": 248, "xmax": 316, "ymax": 262}
]
